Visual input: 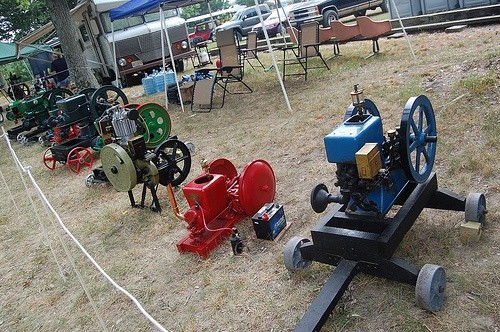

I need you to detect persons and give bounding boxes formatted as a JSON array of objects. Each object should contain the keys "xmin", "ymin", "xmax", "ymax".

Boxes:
[{"xmin": 51, "ymin": 52, "xmax": 71, "ymax": 90}]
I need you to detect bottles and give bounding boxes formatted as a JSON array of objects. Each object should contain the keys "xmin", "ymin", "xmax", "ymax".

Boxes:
[{"xmin": 142, "ymin": 65, "xmax": 176, "ymax": 94}]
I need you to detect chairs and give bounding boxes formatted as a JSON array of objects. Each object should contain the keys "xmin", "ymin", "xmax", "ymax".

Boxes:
[
  {"xmin": 283, "ymin": 16, "xmax": 392, "ymax": 81},
  {"xmin": 190, "ymin": 29, "xmax": 265, "ymax": 113}
]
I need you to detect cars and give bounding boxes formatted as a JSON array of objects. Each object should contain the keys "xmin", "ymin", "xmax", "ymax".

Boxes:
[{"xmin": 250, "ymin": 0, "xmax": 308, "ymax": 40}]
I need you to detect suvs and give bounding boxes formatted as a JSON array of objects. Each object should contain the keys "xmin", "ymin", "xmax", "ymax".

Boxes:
[
  {"xmin": 287, "ymin": 0, "xmax": 390, "ymax": 31},
  {"xmin": 209, "ymin": 3, "xmax": 273, "ymax": 44},
  {"xmin": 187, "ymin": 19, "xmax": 222, "ymax": 47}
]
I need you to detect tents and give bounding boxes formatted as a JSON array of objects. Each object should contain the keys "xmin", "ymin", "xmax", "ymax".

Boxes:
[
  {"xmin": 109, "ymin": 0, "xmax": 418, "ymax": 110},
  {"xmin": 0, "ymin": 41, "xmax": 56, "ymax": 101}
]
